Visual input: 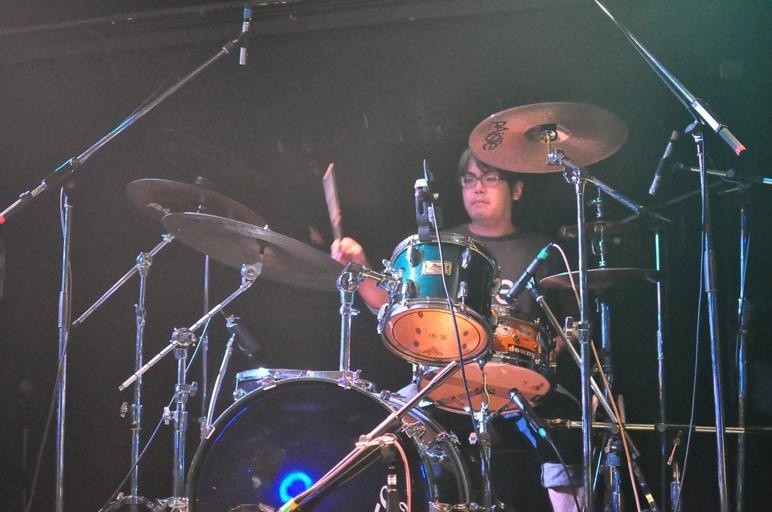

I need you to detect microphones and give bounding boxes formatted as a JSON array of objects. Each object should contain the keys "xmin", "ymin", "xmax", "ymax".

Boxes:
[
  {"xmin": 237, "ymin": 0, "xmax": 256, "ymax": 67},
  {"xmin": 558, "ymin": 225, "xmax": 622, "ymax": 245},
  {"xmin": 423, "ymin": 169, "xmax": 444, "ymax": 231},
  {"xmin": 507, "ymin": 387, "xmax": 552, "ymax": 443},
  {"xmin": 504, "ymin": 242, "xmax": 556, "ymax": 304},
  {"xmin": 225, "ymin": 314, "xmax": 272, "ymax": 368},
  {"xmin": 647, "ymin": 127, "xmax": 681, "ymax": 198}
]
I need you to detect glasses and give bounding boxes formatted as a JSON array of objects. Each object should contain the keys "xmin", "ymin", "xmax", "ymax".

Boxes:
[{"xmin": 461, "ymin": 172, "xmax": 509, "ymax": 190}]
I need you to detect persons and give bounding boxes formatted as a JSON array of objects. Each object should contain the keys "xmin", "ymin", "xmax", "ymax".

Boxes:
[{"xmin": 329, "ymin": 145, "xmax": 588, "ymax": 512}]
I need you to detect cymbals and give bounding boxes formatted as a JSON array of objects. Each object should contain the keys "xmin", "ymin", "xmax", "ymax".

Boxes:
[
  {"xmin": 467, "ymin": 102, "xmax": 628, "ymax": 173},
  {"xmin": 559, "ymin": 218, "xmax": 641, "ymax": 241},
  {"xmin": 539, "ymin": 267, "xmax": 667, "ymax": 297},
  {"xmin": 127, "ymin": 178, "xmax": 270, "ymax": 232},
  {"xmin": 161, "ymin": 213, "xmax": 346, "ymax": 292}
]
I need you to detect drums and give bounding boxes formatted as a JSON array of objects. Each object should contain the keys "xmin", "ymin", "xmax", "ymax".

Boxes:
[
  {"xmin": 232, "ymin": 368, "xmax": 359, "ymax": 400},
  {"xmin": 185, "ymin": 378, "xmax": 470, "ymax": 511},
  {"xmin": 417, "ymin": 310, "xmax": 559, "ymax": 415},
  {"xmin": 379, "ymin": 232, "xmax": 502, "ymax": 367}
]
